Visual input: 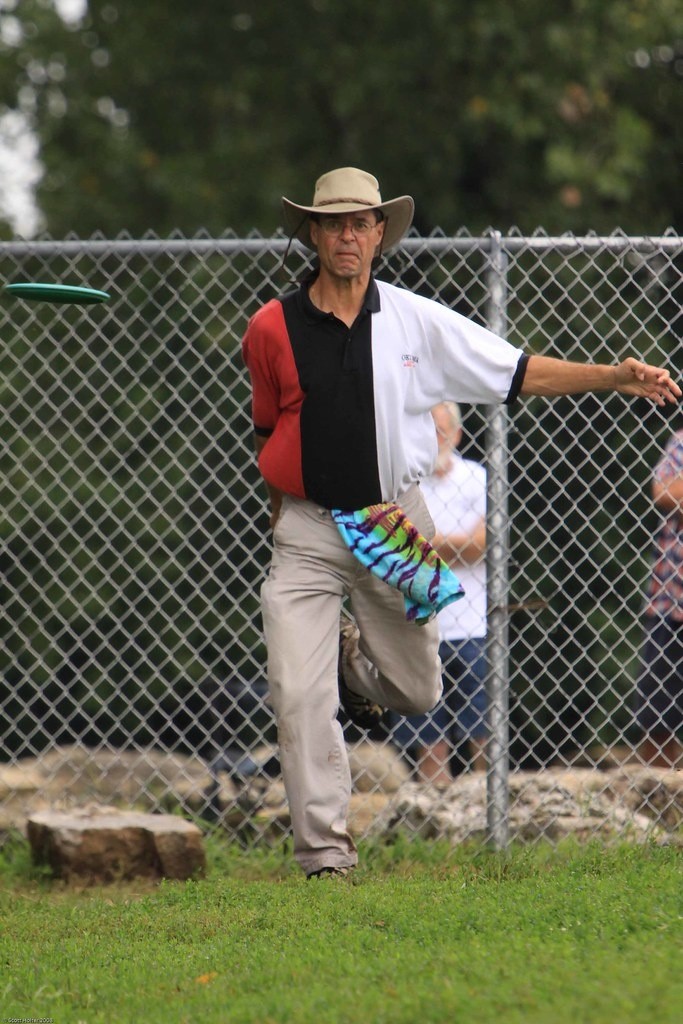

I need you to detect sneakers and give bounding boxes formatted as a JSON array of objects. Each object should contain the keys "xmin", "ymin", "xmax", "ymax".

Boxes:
[
  {"xmin": 308, "ymin": 864, "xmax": 354, "ymax": 887},
  {"xmin": 335, "ymin": 624, "xmax": 383, "ymax": 728}
]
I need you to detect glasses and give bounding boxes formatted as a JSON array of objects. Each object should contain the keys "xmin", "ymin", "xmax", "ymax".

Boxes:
[{"xmin": 314, "ymin": 220, "xmax": 378, "ymax": 236}]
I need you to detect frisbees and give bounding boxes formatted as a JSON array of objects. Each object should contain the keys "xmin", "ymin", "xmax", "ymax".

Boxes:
[{"xmin": 1, "ymin": 281, "xmax": 110, "ymax": 306}]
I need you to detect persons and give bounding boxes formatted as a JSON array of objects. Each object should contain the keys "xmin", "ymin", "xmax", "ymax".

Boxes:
[
  {"xmin": 392, "ymin": 402, "xmax": 487, "ymax": 796},
  {"xmin": 640, "ymin": 431, "xmax": 683, "ymax": 767},
  {"xmin": 241, "ymin": 166, "xmax": 681, "ymax": 883}
]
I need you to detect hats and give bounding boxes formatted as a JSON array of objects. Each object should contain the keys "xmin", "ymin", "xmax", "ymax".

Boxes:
[{"xmin": 281, "ymin": 166, "xmax": 414, "ymax": 258}]
